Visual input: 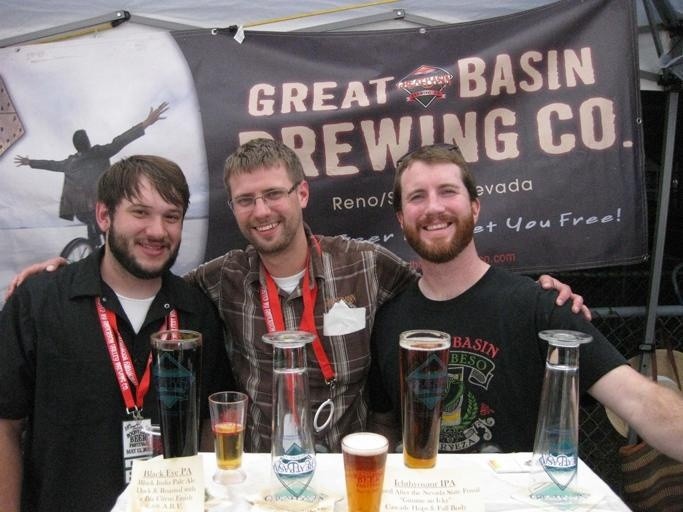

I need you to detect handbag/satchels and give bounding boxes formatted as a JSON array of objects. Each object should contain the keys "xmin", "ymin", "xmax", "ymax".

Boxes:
[{"xmin": 621, "ymin": 346, "xmax": 683, "ymax": 512}]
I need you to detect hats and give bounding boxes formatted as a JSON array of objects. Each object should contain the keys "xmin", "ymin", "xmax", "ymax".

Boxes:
[{"xmin": 606, "ymin": 349, "xmax": 683, "ymax": 438}]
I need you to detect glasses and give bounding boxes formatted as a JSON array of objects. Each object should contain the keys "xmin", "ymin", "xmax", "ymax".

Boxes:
[{"xmin": 227, "ymin": 183, "xmax": 297, "ymax": 212}]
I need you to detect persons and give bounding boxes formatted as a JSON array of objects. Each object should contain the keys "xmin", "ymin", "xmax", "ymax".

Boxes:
[
  {"xmin": 0, "ymin": 152, "xmax": 237, "ymax": 509},
  {"xmin": 13, "ymin": 101, "xmax": 170, "ymax": 249},
  {"xmin": 9, "ymin": 134, "xmax": 596, "ymax": 456},
  {"xmin": 362, "ymin": 142, "xmax": 682, "ymax": 474}
]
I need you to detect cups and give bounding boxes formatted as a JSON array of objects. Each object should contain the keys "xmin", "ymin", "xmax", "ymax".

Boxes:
[
  {"xmin": 341, "ymin": 431, "xmax": 390, "ymax": 512},
  {"xmin": 527, "ymin": 329, "xmax": 593, "ymax": 505},
  {"xmin": 207, "ymin": 390, "xmax": 253, "ymax": 484},
  {"xmin": 262, "ymin": 330, "xmax": 318, "ymax": 474},
  {"xmin": 150, "ymin": 327, "xmax": 204, "ymax": 460},
  {"xmin": 399, "ymin": 328, "xmax": 451, "ymax": 469}
]
